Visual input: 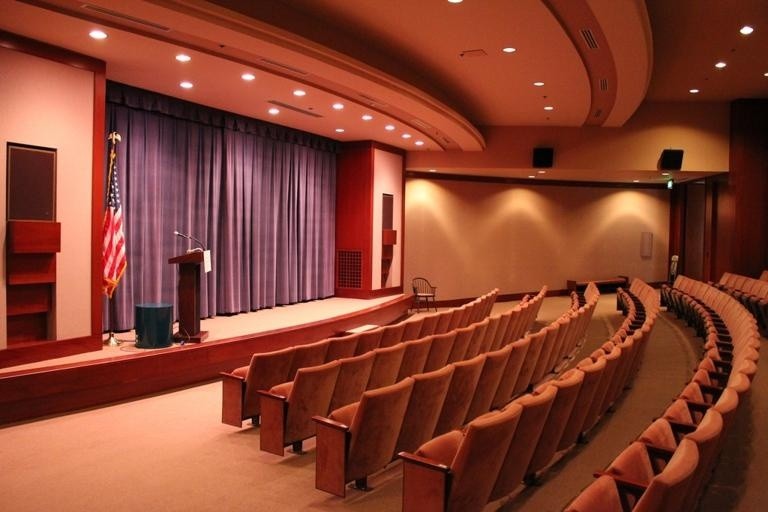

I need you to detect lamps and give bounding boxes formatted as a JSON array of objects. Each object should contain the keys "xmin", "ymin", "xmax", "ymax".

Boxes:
[{"xmin": 666, "ymin": 180, "xmax": 674, "ymax": 190}]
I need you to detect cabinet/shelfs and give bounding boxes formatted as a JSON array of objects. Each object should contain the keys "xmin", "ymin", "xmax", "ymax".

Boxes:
[{"xmin": 169, "ymin": 250, "xmax": 210, "ymax": 344}]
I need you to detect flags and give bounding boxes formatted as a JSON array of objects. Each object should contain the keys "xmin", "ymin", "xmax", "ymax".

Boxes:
[{"xmin": 104, "ymin": 149, "xmax": 127, "ymax": 298}]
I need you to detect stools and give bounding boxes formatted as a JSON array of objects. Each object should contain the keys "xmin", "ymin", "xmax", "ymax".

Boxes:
[{"xmin": 133, "ymin": 303, "xmax": 173, "ymax": 348}]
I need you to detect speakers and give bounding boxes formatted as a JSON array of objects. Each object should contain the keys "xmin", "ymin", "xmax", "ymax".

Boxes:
[
  {"xmin": 532, "ymin": 147, "xmax": 559, "ymax": 168},
  {"xmin": 657, "ymin": 149, "xmax": 684, "ymax": 171}
]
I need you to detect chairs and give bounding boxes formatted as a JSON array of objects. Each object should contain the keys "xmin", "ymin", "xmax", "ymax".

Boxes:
[
  {"xmin": 529, "ymin": 321, "xmax": 560, "ymax": 385},
  {"xmin": 533, "ymin": 359, "xmax": 607, "ymax": 451},
  {"xmin": 434, "ymin": 354, "xmax": 487, "ymax": 439},
  {"xmin": 503, "ymin": 372, "xmax": 586, "ymax": 483},
  {"xmin": 702, "ymin": 298, "xmax": 737, "ymax": 326},
  {"xmin": 485, "ymin": 290, "xmax": 496, "ymax": 315},
  {"xmin": 671, "ymin": 279, "xmax": 696, "ymax": 316},
  {"xmin": 676, "ymin": 281, "xmax": 702, "ymax": 319},
  {"xmin": 611, "ymin": 319, "xmax": 655, "ymax": 371},
  {"xmin": 705, "ymin": 310, "xmax": 750, "ymax": 343},
  {"xmin": 594, "ymin": 407, "xmax": 723, "ymax": 509},
  {"xmin": 219, "ymin": 346, "xmax": 296, "ymax": 428},
  {"xmin": 501, "ymin": 307, "xmax": 522, "ymax": 346},
  {"xmin": 750, "ymin": 282, "xmax": 768, "ymax": 330},
  {"xmin": 734, "ymin": 278, "xmax": 757, "ymax": 302},
  {"xmin": 327, "ymin": 334, "xmax": 361, "ymax": 363},
  {"xmin": 288, "ymin": 339, "xmax": 330, "ymax": 382},
  {"xmin": 630, "ymin": 387, "xmax": 740, "ymax": 472},
  {"xmin": 424, "ymin": 330, "xmax": 457, "ymax": 373},
  {"xmin": 602, "ymin": 324, "xmax": 650, "ymax": 382},
  {"xmin": 722, "ymin": 274, "xmax": 740, "ymax": 293},
  {"xmin": 327, "ymin": 351, "xmax": 376, "ymax": 416},
  {"xmin": 395, "ymin": 364, "xmax": 456, "ymax": 460},
  {"xmin": 419, "ymin": 313, "xmax": 441, "ymax": 338},
  {"xmin": 448, "ymin": 324, "xmax": 475, "ymax": 364},
  {"xmin": 469, "ymin": 298, "xmax": 482, "ymax": 324},
  {"xmin": 257, "ymin": 360, "xmax": 341, "ymax": 457},
  {"xmin": 695, "ymin": 291, "xmax": 726, "ymax": 327},
  {"xmin": 758, "ymin": 270, "xmax": 768, "ymax": 281},
  {"xmin": 435, "ymin": 309, "xmax": 454, "ymax": 334},
  {"xmin": 464, "ymin": 318, "xmax": 490, "ymax": 360},
  {"xmin": 403, "ymin": 317, "xmax": 424, "ymax": 341},
  {"xmin": 490, "ymin": 287, "xmax": 500, "ymax": 313},
  {"xmin": 672, "ymin": 359, "xmax": 758, "ymax": 415},
  {"xmin": 727, "ymin": 276, "xmax": 747, "ymax": 297},
  {"xmin": 491, "ymin": 338, "xmax": 532, "ymax": 409},
  {"xmin": 699, "ymin": 294, "xmax": 731, "ymax": 327},
  {"xmin": 354, "ymin": 326, "xmax": 385, "ymax": 356},
  {"xmin": 311, "ymin": 377, "xmax": 414, "ymax": 499},
  {"xmin": 758, "ymin": 289, "xmax": 768, "ymax": 338},
  {"xmin": 396, "ymin": 404, "xmax": 520, "ymax": 512},
  {"xmin": 463, "ymin": 385, "xmax": 559, "ymax": 503},
  {"xmin": 483, "ymin": 292, "xmax": 492, "ymax": 316},
  {"xmin": 661, "ymin": 274, "xmax": 685, "ymax": 313},
  {"xmin": 558, "ymin": 312, "xmax": 578, "ymax": 364},
  {"xmin": 477, "ymin": 295, "xmax": 487, "ymax": 320},
  {"xmin": 616, "ymin": 277, "xmax": 661, "ymax": 325},
  {"xmin": 491, "ymin": 310, "xmax": 513, "ymax": 351},
  {"xmin": 699, "ymin": 329, "xmax": 761, "ymax": 368},
  {"xmin": 411, "ymin": 277, "xmax": 439, "ymax": 314},
  {"xmin": 521, "ymin": 285, "xmax": 548, "ymax": 330},
  {"xmin": 691, "ymin": 289, "xmax": 720, "ymax": 325},
  {"xmin": 557, "ymin": 347, "xmax": 621, "ymax": 425},
  {"xmin": 693, "ymin": 338, "xmax": 761, "ymax": 382},
  {"xmin": 478, "ymin": 314, "xmax": 502, "ymax": 355},
  {"xmin": 686, "ymin": 347, "xmax": 759, "ymax": 390},
  {"xmin": 449, "ymin": 305, "xmax": 465, "ymax": 330},
  {"xmin": 681, "ymin": 284, "xmax": 709, "ymax": 321},
  {"xmin": 545, "ymin": 317, "xmax": 570, "ymax": 376},
  {"xmin": 576, "ymin": 337, "xmax": 634, "ymax": 411},
  {"xmin": 512, "ymin": 302, "xmax": 529, "ymax": 339},
  {"xmin": 569, "ymin": 281, "xmax": 600, "ymax": 334},
  {"xmin": 653, "ymin": 373, "xmax": 751, "ymax": 440},
  {"xmin": 367, "ymin": 342, "xmax": 407, "ymax": 390},
  {"xmin": 715, "ymin": 271, "xmax": 731, "ymax": 289},
  {"xmin": 686, "ymin": 286, "xmax": 714, "ymax": 325},
  {"xmin": 381, "ymin": 322, "xmax": 406, "ymax": 348},
  {"xmin": 742, "ymin": 280, "xmax": 764, "ymax": 311},
  {"xmin": 459, "ymin": 301, "xmax": 475, "ymax": 327},
  {"xmin": 705, "ymin": 303, "xmax": 742, "ymax": 332},
  {"xmin": 665, "ymin": 277, "xmax": 690, "ymax": 315},
  {"xmin": 707, "ymin": 314, "xmax": 753, "ymax": 348},
  {"xmin": 465, "ymin": 344, "xmax": 513, "ymax": 425},
  {"xmin": 590, "ymin": 329, "xmax": 642, "ymax": 399},
  {"xmin": 706, "ymin": 322, "xmax": 757, "ymax": 339},
  {"xmin": 513, "ymin": 328, "xmax": 547, "ymax": 395},
  {"xmin": 397, "ymin": 335, "xmax": 435, "ymax": 383},
  {"xmin": 562, "ymin": 438, "xmax": 698, "ymax": 512}
]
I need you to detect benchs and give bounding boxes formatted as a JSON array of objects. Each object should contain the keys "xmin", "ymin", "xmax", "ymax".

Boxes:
[{"xmin": 567, "ymin": 276, "xmax": 630, "ymax": 296}]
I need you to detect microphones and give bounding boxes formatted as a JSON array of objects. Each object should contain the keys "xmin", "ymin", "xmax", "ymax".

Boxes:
[{"xmin": 173, "ymin": 230, "xmax": 187, "ymax": 240}]
